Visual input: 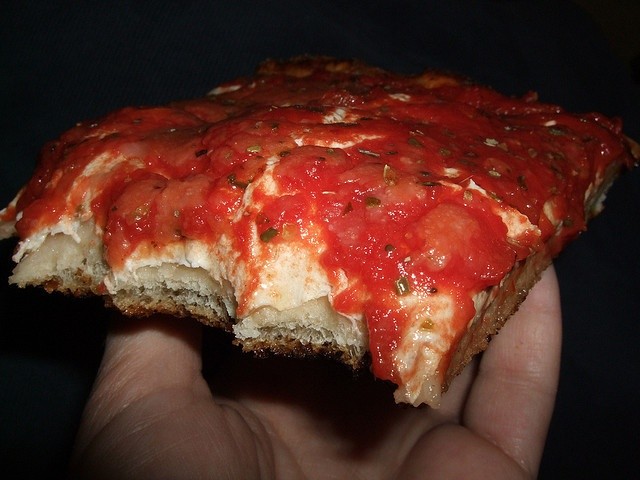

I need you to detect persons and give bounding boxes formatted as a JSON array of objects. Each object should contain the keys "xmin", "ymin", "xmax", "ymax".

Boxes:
[{"xmin": 67, "ymin": 262, "xmax": 562, "ymax": 480}]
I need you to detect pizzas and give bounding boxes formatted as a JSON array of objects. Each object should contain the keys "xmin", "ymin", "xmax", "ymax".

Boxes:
[{"xmin": 2, "ymin": 49, "xmax": 637, "ymax": 406}]
